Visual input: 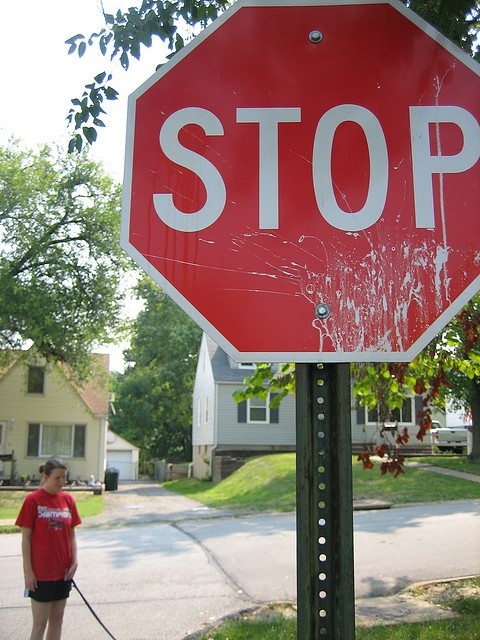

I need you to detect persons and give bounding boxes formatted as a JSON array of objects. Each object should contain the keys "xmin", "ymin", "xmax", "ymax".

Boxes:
[{"xmin": 14, "ymin": 457, "xmax": 81, "ymax": 640}]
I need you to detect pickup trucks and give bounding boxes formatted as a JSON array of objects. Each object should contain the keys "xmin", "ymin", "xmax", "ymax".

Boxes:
[{"xmin": 430, "ymin": 420, "xmax": 468, "ymax": 453}]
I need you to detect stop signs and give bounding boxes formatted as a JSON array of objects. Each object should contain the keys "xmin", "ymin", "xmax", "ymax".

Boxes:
[{"xmin": 119, "ymin": 0, "xmax": 480, "ymax": 363}]
